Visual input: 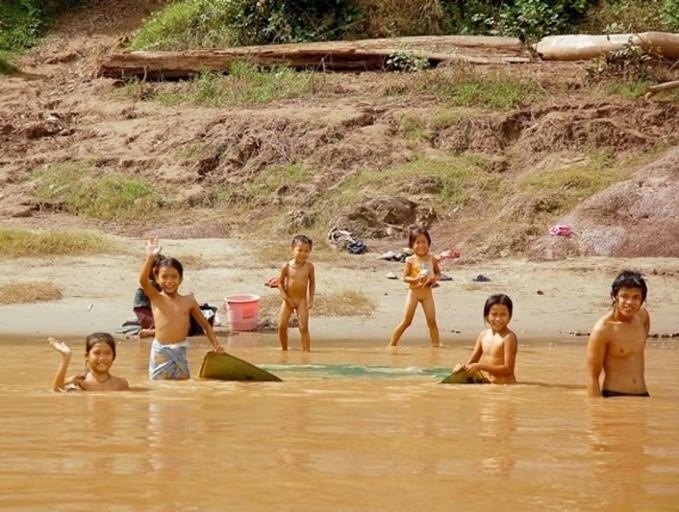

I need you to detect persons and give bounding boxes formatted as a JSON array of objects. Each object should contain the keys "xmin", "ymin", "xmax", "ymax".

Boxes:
[
  {"xmin": 454, "ymin": 295, "xmax": 517, "ymax": 385},
  {"xmin": 124, "ymin": 254, "xmax": 169, "ymax": 337},
  {"xmin": 48, "ymin": 332, "xmax": 128, "ymax": 392},
  {"xmin": 139, "ymin": 239, "xmax": 225, "ymax": 379},
  {"xmin": 587, "ymin": 271, "xmax": 650, "ymax": 397},
  {"xmin": 391, "ymin": 228, "xmax": 443, "ymax": 347},
  {"xmin": 278, "ymin": 234, "xmax": 315, "ymax": 352}
]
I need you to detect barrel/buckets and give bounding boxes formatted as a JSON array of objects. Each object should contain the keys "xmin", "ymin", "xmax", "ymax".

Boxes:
[{"xmin": 224, "ymin": 294, "xmax": 261, "ymax": 332}]
